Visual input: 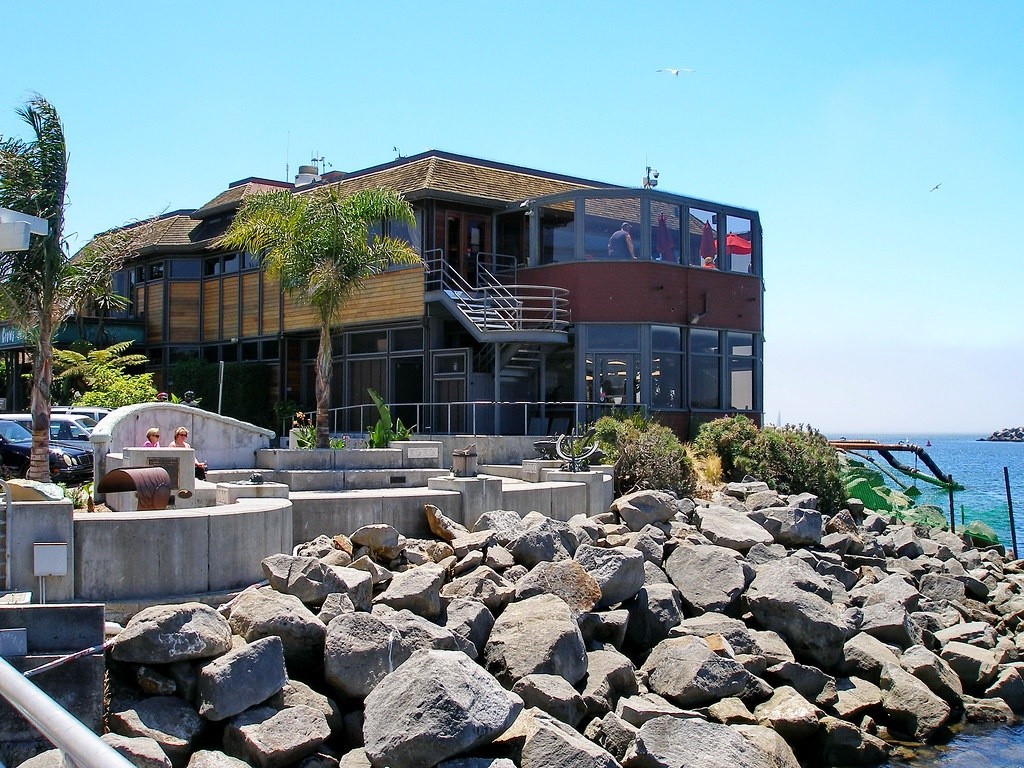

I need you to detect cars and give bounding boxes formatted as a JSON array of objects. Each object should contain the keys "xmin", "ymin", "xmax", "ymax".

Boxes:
[{"xmin": 0, "ymin": 421, "xmax": 94, "ymax": 483}]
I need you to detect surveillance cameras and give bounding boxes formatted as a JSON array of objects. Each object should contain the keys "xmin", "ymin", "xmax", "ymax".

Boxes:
[{"xmin": 653, "ymin": 172, "xmax": 659, "ymax": 178}]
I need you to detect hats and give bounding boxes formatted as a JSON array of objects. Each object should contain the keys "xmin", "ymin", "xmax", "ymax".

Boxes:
[{"xmin": 620, "ymin": 222, "xmax": 633, "ymax": 230}]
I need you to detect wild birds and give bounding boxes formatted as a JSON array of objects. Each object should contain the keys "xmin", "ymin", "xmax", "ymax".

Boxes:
[
  {"xmin": 656, "ymin": 68, "xmax": 696, "ymax": 77},
  {"xmin": 930, "ymin": 182, "xmax": 942, "ymax": 192}
]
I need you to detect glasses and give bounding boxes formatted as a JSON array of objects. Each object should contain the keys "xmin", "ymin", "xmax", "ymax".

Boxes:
[
  {"xmin": 177, "ymin": 433, "xmax": 188, "ymax": 437},
  {"xmin": 151, "ymin": 434, "xmax": 160, "ymax": 438}
]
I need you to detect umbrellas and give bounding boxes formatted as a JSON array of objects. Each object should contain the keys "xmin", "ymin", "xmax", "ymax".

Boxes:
[
  {"xmin": 657, "ymin": 213, "xmax": 674, "ymax": 261},
  {"xmin": 714, "ymin": 230, "xmax": 751, "ymax": 255},
  {"xmin": 701, "ymin": 220, "xmax": 716, "ymax": 260}
]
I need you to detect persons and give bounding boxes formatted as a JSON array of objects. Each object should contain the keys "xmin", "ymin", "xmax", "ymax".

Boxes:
[
  {"xmin": 608, "ymin": 222, "xmax": 637, "ymax": 260},
  {"xmin": 703, "ymin": 257, "xmax": 715, "ymax": 268},
  {"xmin": 144, "ymin": 428, "xmax": 160, "ymax": 447},
  {"xmin": 168, "ymin": 427, "xmax": 207, "ymax": 481},
  {"xmin": 58, "ymin": 422, "xmax": 73, "ymax": 439}
]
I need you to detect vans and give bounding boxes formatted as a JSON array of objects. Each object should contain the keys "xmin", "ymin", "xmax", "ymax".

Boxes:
[{"xmin": 0, "ymin": 415, "xmax": 99, "ymax": 452}]
